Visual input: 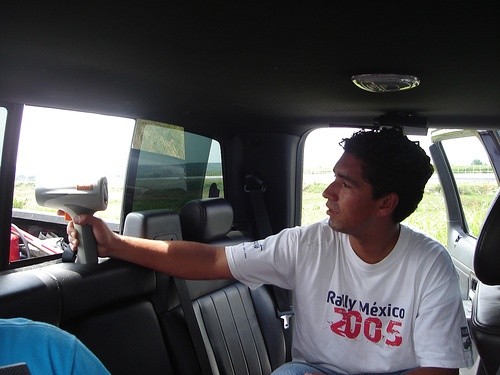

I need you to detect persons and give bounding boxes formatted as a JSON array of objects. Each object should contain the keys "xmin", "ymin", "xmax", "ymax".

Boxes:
[
  {"xmin": 0, "ymin": 318, "xmax": 110, "ymax": 375},
  {"xmin": 58, "ymin": 125, "xmax": 478, "ymax": 375}
]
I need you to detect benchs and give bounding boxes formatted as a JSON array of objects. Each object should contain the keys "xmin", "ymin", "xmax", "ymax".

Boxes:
[{"xmin": 1, "ymin": 201, "xmax": 287, "ymax": 375}]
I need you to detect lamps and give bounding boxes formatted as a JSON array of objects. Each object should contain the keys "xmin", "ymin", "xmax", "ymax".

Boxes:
[{"xmin": 349, "ymin": 73, "xmax": 420, "ymax": 93}]
